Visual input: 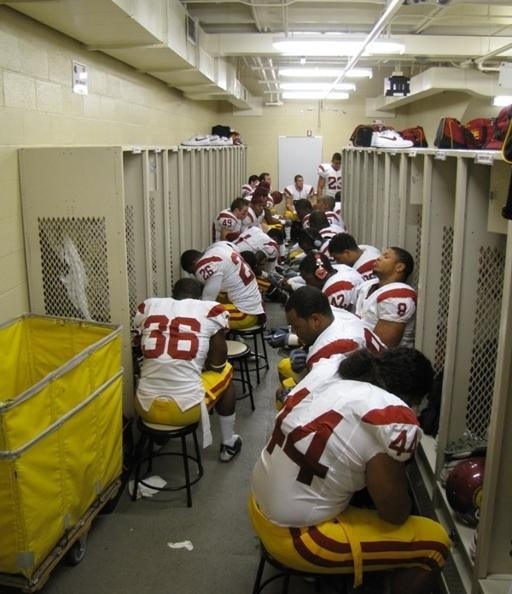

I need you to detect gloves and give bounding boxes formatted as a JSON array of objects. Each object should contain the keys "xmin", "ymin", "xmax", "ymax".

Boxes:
[
  {"xmin": 263, "ymin": 327, "xmax": 307, "ymax": 373},
  {"xmin": 267, "ymin": 259, "xmax": 302, "ymax": 288}
]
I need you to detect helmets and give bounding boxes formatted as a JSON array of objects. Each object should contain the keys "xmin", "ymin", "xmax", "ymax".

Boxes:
[{"xmin": 446, "ymin": 457, "xmax": 484, "ymax": 528}]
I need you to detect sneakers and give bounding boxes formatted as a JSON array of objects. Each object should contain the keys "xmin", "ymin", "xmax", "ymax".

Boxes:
[
  {"xmin": 371, "ymin": 130, "xmax": 414, "ymax": 148},
  {"xmin": 220, "ymin": 434, "xmax": 242, "ymax": 462},
  {"xmin": 181, "ymin": 134, "xmax": 233, "ymax": 146}
]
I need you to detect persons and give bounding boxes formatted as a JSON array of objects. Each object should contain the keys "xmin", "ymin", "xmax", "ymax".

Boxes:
[
  {"xmin": 317, "ymin": 152, "xmax": 343, "ymax": 198},
  {"xmin": 129, "ymin": 277, "xmax": 242, "ymax": 464},
  {"xmin": 248, "ymin": 346, "xmax": 452, "ymax": 594},
  {"xmin": 178, "ymin": 172, "xmax": 420, "ymax": 348},
  {"xmin": 283, "ymin": 287, "xmax": 390, "ymax": 408}
]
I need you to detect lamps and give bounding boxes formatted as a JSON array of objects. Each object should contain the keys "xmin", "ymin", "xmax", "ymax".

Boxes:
[{"xmin": 275, "ymin": 36, "xmax": 405, "ymax": 102}]
